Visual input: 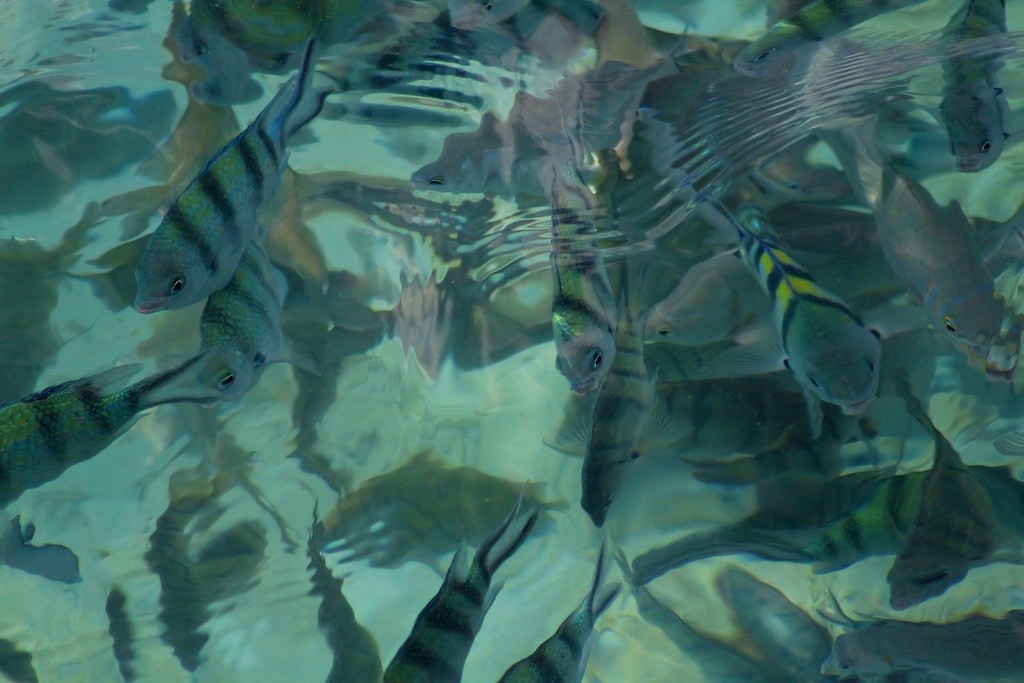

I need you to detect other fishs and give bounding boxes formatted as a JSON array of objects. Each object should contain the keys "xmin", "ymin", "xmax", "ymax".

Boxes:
[{"xmin": 0, "ymin": 0, "xmax": 1023, "ymax": 683}]
